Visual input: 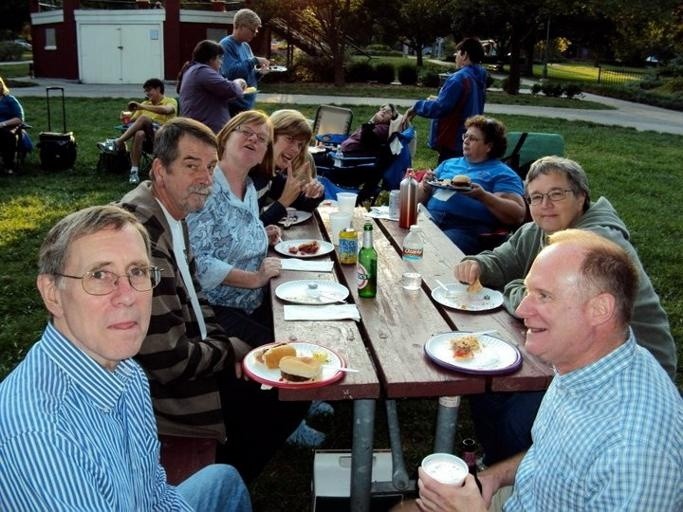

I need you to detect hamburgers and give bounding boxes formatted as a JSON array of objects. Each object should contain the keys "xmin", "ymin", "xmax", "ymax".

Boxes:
[
  {"xmin": 253, "ymin": 342, "xmax": 297, "ymax": 370},
  {"xmin": 451, "ymin": 174, "xmax": 470, "ymax": 188},
  {"xmin": 279, "ymin": 355, "xmax": 319, "ymax": 385}
]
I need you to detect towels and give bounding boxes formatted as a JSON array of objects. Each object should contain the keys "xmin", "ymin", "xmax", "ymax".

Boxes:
[
  {"xmin": 270, "ymin": 252, "xmax": 337, "ymax": 275},
  {"xmin": 281, "ymin": 300, "xmax": 360, "ymax": 327}
]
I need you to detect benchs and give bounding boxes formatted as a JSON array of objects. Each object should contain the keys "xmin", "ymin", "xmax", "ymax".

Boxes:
[{"xmin": 155, "ymin": 432, "xmax": 216, "ymax": 488}]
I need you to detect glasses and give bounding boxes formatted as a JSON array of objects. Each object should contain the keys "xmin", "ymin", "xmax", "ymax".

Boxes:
[
  {"xmin": 461, "ymin": 133, "xmax": 484, "ymax": 142},
  {"xmin": 52, "ymin": 266, "xmax": 164, "ymax": 295},
  {"xmin": 526, "ymin": 189, "xmax": 573, "ymax": 205},
  {"xmin": 236, "ymin": 125, "xmax": 269, "ymax": 144}
]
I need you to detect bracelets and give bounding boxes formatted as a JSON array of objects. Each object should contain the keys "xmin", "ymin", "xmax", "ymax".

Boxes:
[{"xmin": 0, "ymin": 120, "xmax": 9, "ymax": 128}]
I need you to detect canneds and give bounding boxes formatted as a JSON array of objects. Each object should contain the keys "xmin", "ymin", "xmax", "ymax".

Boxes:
[
  {"xmin": 339, "ymin": 228, "xmax": 358, "ymax": 265},
  {"xmin": 389, "ymin": 189, "xmax": 401, "ymax": 221}
]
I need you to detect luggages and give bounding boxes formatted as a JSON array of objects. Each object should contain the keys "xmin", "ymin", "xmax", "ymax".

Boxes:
[{"xmin": 36, "ymin": 87, "xmax": 76, "ymax": 170}]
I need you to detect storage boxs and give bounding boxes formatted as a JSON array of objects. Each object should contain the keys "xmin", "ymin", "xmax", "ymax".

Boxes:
[{"xmin": 309, "ymin": 449, "xmax": 403, "ymax": 512}]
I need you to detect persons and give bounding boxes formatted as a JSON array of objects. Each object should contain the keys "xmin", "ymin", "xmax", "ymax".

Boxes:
[
  {"xmin": 415, "ymin": 113, "xmax": 528, "ymax": 255},
  {"xmin": 178, "ymin": 40, "xmax": 248, "ymax": 136},
  {"xmin": 390, "ymin": 229, "xmax": 683, "ymax": 512},
  {"xmin": 314, "ymin": 103, "xmax": 397, "ymax": 176},
  {"xmin": 401, "ymin": 37, "xmax": 492, "ymax": 169},
  {"xmin": 181, "ymin": 108, "xmax": 335, "ymax": 451},
  {"xmin": 0, "ymin": 203, "xmax": 252, "ymax": 511},
  {"xmin": 94, "ymin": 78, "xmax": 179, "ymax": 186},
  {"xmin": 217, "ymin": 9, "xmax": 270, "ymax": 110},
  {"xmin": 451, "ymin": 154, "xmax": 678, "ymax": 470},
  {"xmin": 0, "ymin": 76, "xmax": 33, "ymax": 175},
  {"xmin": 118, "ymin": 117, "xmax": 313, "ymax": 486}
]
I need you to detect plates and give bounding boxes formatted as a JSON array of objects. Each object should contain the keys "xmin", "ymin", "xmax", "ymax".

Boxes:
[
  {"xmin": 266, "ymin": 206, "xmax": 311, "ymax": 227},
  {"xmin": 258, "ymin": 65, "xmax": 287, "ymax": 73},
  {"xmin": 425, "ymin": 177, "xmax": 473, "ymax": 192},
  {"xmin": 430, "ymin": 283, "xmax": 505, "ymax": 313},
  {"xmin": 423, "ymin": 332, "xmax": 523, "ymax": 374},
  {"xmin": 271, "ymin": 237, "xmax": 333, "ymax": 257},
  {"xmin": 274, "ymin": 278, "xmax": 348, "ymax": 304},
  {"xmin": 241, "ymin": 339, "xmax": 346, "ymax": 390},
  {"xmin": 243, "ymin": 89, "xmax": 260, "ymax": 96}
]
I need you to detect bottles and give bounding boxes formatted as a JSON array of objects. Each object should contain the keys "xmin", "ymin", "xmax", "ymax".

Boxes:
[
  {"xmin": 398, "ymin": 168, "xmax": 417, "ymax": 227},
  {"xmin": 459, "ymin": 438, "xmax": 481, "ymax": 496},
  {"xmin": 332, "ymin": 145, "xmax": 343, "ymax": 167},
  {"xmin": 354, "ymin": 224, "xmax": 378, "ymax": 298}
]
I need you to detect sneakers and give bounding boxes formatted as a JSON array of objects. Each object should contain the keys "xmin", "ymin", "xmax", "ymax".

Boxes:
[
  {"xmin": 129, "ymin": 171, "xmax": 140, "ymax": 185},
  {"xmin": 97, "ymin": 139, "xmax": 119, "ymax": 156},
  {"xmin": 286, "ymin": 400, "xmax": 334, "ymax": 448}
]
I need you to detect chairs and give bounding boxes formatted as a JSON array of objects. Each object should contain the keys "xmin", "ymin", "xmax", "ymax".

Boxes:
[
  {"xmin": 499, "ymin": 132, "xmax": 564, "ymax": 180},
  {"xmin": 304, "ymin": 104, "xmax": 415, "ymax": 209}
]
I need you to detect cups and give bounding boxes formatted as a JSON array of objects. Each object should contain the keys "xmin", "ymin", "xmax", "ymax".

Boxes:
[
  {"xmin": 336, "ymin": 192, "xmax": 357, "ymax": 214},
  {"xmin": 328, "ymin": 212, "xmax": 350, "ymax": 247},
  {"xmin": 420, "ymin": 453, "xmax": 469, "ymax": 486},
  {"xmin": 399, "ymin": 224, "xmax": 424, "ymax": 291}
]
